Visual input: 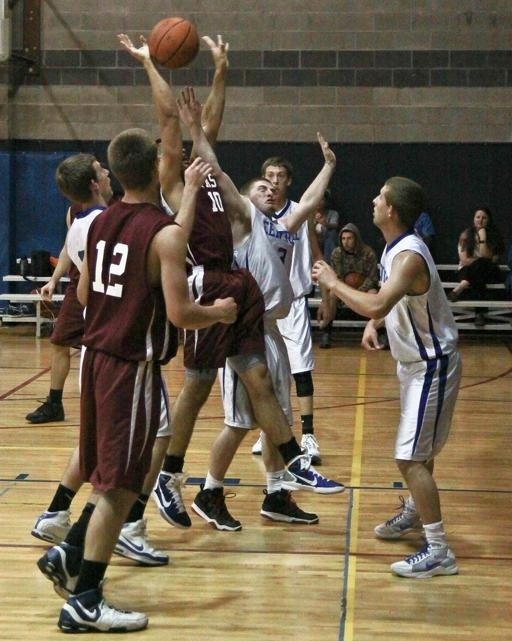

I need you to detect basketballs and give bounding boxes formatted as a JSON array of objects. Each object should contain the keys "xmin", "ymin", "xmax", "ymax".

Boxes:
[
  {"xmin": 344, "ymin": 271, "xmax": 363, "ymax": 288},
  {"xmin": 146, "ymin": 15, "xmax": 202, "ymax": 70}
]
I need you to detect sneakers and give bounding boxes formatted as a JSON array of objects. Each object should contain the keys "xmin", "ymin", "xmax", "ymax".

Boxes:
[
  {"xmin": 372, "ymin": 495, "xmax": 425, "ymax": 539},
  {"xmin": 31, "ymin": 508, "xmax": 73, "ymax": 544},
  {"xmin": 281, "ymin": 448, "xmax": 344, "ymax": 494},
  {"xmin": 259, "ymin": 489, "xmax": 319, "ymax": 525},
  {"xmin": 318, "ymin": 333, "xmax": 330, "ymax": 347},
  {"xmin": 299, "ymin": 433, "xmax": 322, "ymax": 465},
  {"xmin": 149, "ymin": 471, "xmax": 191, "ymax": 530},
  {"xmin": 474, "ymin": 314, "xmax": 484, "ymax": 326},
  {"xmin": 251, "ymin": 430, "xmax": 262, "ymax": 454},
  {"xmin": 447, "ymin": 291, "xmax": 456, "ymax": 302},
  {"xmin": 25, "ymin": 400, "xmax": 65, "ymax": 424},
  {"xmin": 390, "ymin": 545, "xmax": 458, "ymax": 579},
  {"xmin": 112, "ymin": 517, "xmax": 169, "ymax": 566},
  {"xmin": 36, "ymin": 540, "xmax": 81, "ymax": 601},
  {"xmin": 57, "ymin": 595, "xmax": 148, "ymax": 633},
  {"xmin": 191, "ymin": 482, "xmax": 243, "ymax": 532}
]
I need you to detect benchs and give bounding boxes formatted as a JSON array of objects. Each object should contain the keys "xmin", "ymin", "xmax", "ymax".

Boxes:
[{"xmin": 0, "ymin": 266, "xmax": 512, "ymax": 344}]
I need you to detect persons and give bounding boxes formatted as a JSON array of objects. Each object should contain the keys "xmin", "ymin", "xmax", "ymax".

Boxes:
[
  {"xmin": 414, "ymin": 213, "xmax": 434, "ymax": 251},
  {"xmin": 36, "ymin": 133, "xmax": 238, "ymax": 635},
  {"xmin": 169, "ymin": 83, "xmax": 337, "ymax": 532},
  {"xmin": 116, "ymin": 30, "xmax": 347, "ymax": 530},
  {"xmin": 446, "ymin": 205, "xmax": 503, "ymax": 329},
  {"xmin": 24, "ymin": 197, "xmax": 119, "ymax": 425},
  {"xmin": 312, "ymin": 175, "xmax": 463, "ymax": 582},
  {"xmin": 250, "ymin": 152, "xmax": 335, "ymax": 469},
  {"xmin": 319, "ymin": 222, "xmax": 389, "ymax": 352},
  {"xmin": 30, "ymin": 151, "xmax": 174, "ymax": 567},
  {"xmin": 314, "ymin": 194, "xmax": 339, "ymax": 266}
]
